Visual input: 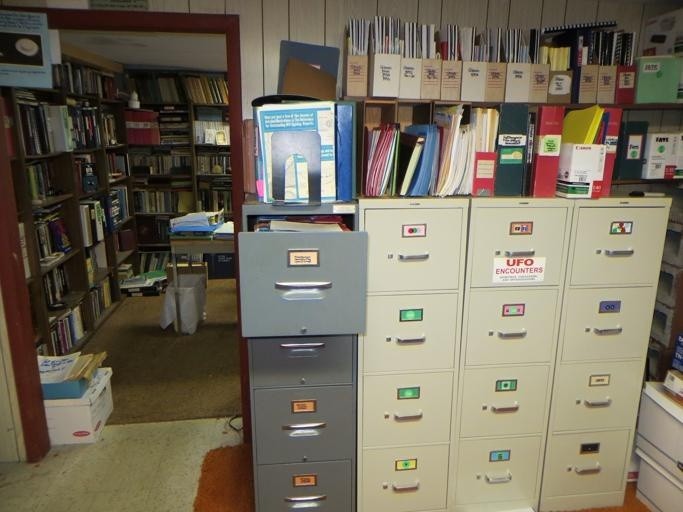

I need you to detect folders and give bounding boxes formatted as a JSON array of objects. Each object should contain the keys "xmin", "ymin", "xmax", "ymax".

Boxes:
[
  {"xmin": 494, "ymin": 104, "xmax": 528, "ymax": 197},
  {"xmin": 337, "ymin": 103, "xmax": 353, "ymax": 204},
  {"xmin": 531, "ymin": 104, "xmax": 565, "ymax": 197},
  {"xmin": 603, "ymin": 107, "xmax": 622, "ymax": 198}
]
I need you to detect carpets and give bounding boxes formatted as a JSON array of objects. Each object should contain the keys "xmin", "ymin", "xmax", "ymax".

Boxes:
[
  {"xmin": 193, "ymin": 441, "xmax": 254, "ymax": 512},
  {"xmin": 77, "ymin": 276, "xmax": 243, "ymax": 425}
]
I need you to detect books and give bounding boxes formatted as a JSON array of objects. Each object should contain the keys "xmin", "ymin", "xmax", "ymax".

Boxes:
[
  {"xmin": 251, "ymin": 213, "xmax": 355, "ymax": 236},
  {"xmin": 2, "ymin": 57, "xmax": 234, "ymax": 402},
  {"xmin": 364, "ymin": 105, "xmax": 683, "ymax": 198},
  {"xmin": 346, "ymin": 16, "xmax": 636, "ymax": 71},
  {"xmin": 642, "ymin": 337, "xmax": 682, "ymax": 423},
  {"xmin": 251, "ymin": 99, "xmax": 357, "ymax": 207}
]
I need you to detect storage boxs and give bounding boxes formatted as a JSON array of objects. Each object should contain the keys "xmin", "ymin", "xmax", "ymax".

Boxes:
[{"xmin": 43, "ymin": 367, "xmax": 114, "ymax": 446}]
